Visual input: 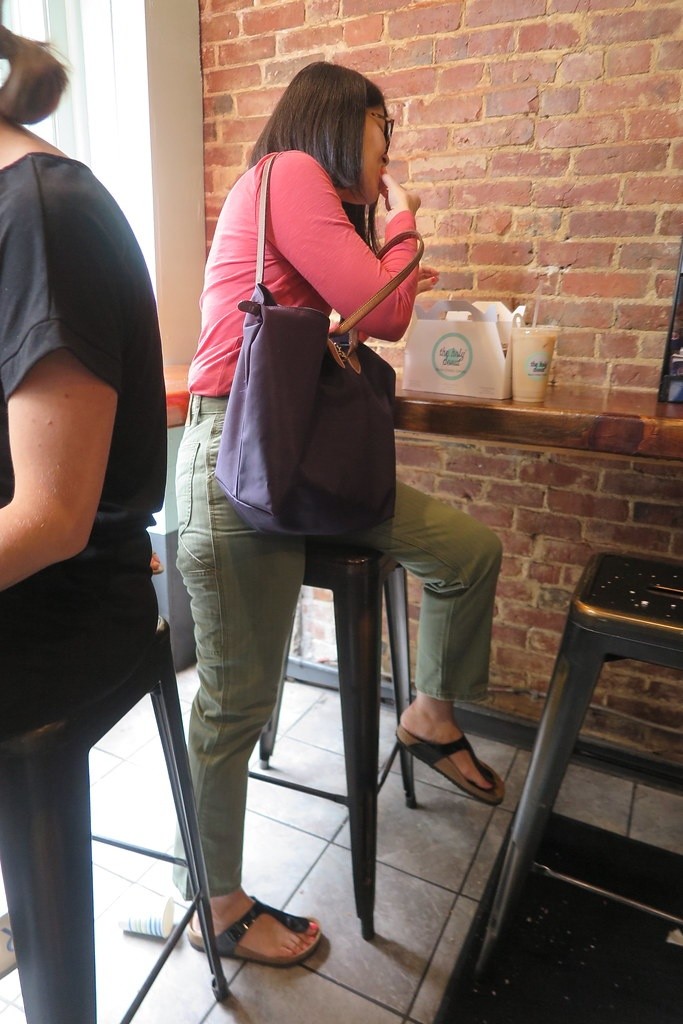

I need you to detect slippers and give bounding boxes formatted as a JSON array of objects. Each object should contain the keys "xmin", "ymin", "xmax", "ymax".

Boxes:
[
  {"xmin": 185, "ymin": 895, "xmax": 322, "ymax": 967},
  {"xmin": 394, "ymin": 722, "xmax": 506, "ymax": 805}
]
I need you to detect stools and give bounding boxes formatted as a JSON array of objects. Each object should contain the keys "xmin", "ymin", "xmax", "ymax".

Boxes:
[
  {"xmin": 260, "ymin": 536, "xmax": 420, "ymax": 940},
  {"xmin": 471, "ymin": 550, "xmax": 683, "ymax": 997},
  {"xmin": 0, "ymin": 617, "xmax": 228, "ymax": 1023}
]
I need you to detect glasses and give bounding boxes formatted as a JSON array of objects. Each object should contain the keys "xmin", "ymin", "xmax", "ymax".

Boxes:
[{"xmin": 370, "ymin": 111, "xmax": 395, "ymax": 142}]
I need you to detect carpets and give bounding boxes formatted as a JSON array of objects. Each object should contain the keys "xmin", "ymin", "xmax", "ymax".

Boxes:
[{"xmin": 432, "ymin": 802, "xmax": 683, "ymax": 1024}]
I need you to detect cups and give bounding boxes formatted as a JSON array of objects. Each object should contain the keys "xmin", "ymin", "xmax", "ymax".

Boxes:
[{"xmin": 510, "ymin": 324, "xmax": 560, "ymax": 403}]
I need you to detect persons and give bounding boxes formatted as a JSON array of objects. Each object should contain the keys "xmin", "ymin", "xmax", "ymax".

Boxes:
[
  {"xmin": 175, "ymin": 63, "xmax": 504, "ymax": 966},
  {"xmin": 0, "ymin": 24, "xmax": 167, "ymax": 729}
]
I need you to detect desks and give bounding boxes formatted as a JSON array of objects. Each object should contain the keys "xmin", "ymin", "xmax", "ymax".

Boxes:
[{"xmin": 163, "ymin": 363, "xmax": 683, "ymax": 466}]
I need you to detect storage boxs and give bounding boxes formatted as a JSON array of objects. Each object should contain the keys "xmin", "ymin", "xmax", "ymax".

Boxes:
[{"xmin": 402, "ymin": 298, "xmax": 525, "ymax": 401}]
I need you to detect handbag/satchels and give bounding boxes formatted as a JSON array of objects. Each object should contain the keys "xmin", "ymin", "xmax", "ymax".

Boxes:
[{"xmin": 211, "ymin": 154, "xmax": 426, "ymax": 541}]
